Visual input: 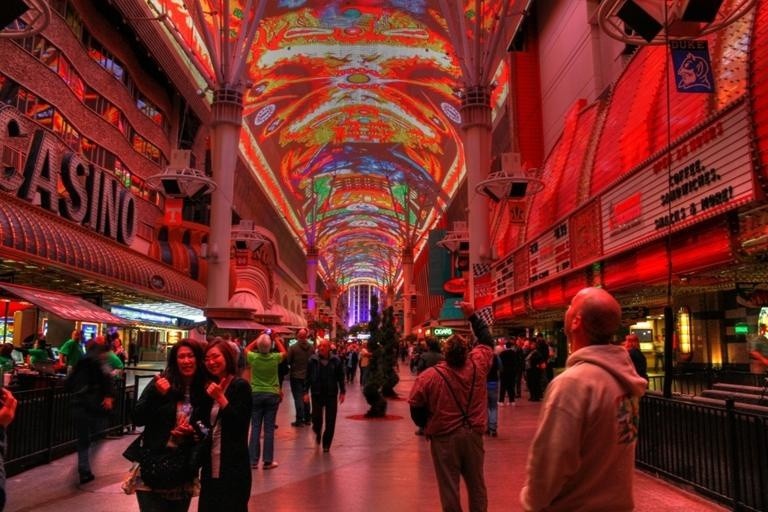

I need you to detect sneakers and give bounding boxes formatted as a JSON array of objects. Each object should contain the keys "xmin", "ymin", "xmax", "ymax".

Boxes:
[{"xmin": 248, "ymin": 418, "xmax": 331, "ymax": 471}]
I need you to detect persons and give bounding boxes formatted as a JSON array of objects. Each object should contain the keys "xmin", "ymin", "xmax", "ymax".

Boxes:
[
  {"xmin": 624, "ymin": 334, "xmax": 648, "ymax": 384},
  {"xmin": 400, "ymin": 303, "xmax": 552, "ymax": 512},
  {"xmin": 129, "ymin": 329, "xmax": 345, "ymax": 512},
  {"xmin": 0, "ymin": 328, "xmax": 139, "ymax": 512},
  {"xmin": 653, "ymin": 335, "xmax": 665, "ymax": 372},
  {"xmin": 520, "ymin": 288, "xmax": 650, "ymax": 512},
  {"xmin": 752, "ymin": 323, "xmax": 768, "ymax": 366},
  {"xmin": 344, "ymin": 340, "xmax": 374, "ymax": 384}
]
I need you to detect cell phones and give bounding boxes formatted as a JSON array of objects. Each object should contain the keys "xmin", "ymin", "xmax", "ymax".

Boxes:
[{"xmin": 266, "ymin": 329, "xmax": 272, "ymax": 334}]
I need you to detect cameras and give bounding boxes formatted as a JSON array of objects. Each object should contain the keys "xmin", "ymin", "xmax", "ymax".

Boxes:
[{"xmin": 195, "ymin": 420, "xmax": 210, "ymax": 440}]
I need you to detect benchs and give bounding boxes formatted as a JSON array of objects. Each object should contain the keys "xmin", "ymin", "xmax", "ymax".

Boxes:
[{"xmin": 645, "ymin": 370, "xmax": 768, "ymax": 462}]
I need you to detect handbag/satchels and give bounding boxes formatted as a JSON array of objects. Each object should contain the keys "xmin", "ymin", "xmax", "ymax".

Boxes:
[{"xmin": 137, "ymin": 439, "xmax": 194, "ymax": 491}]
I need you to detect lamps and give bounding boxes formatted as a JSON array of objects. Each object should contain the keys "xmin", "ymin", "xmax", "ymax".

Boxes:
[
  {"xmin": 437, "ymin": 145, "xmax": 469, "ymax": 253},
  {"xmin": 474, "ymin": 1, "xmax": 544, "ymax": 205},
  {"xmin": 2, "ymin": 1, "xmax": 51, "ymax": 39},
  {"xmin": 598, "ymin": 0, "xmax": 757, "ymax": 46},
  {"xmin": 230, "ymin": 0, "xmax": 270, "ymax": 253},
  {"xmin": 146, "ymin": 0, "xmax": 216, "ymax": 201}
]
